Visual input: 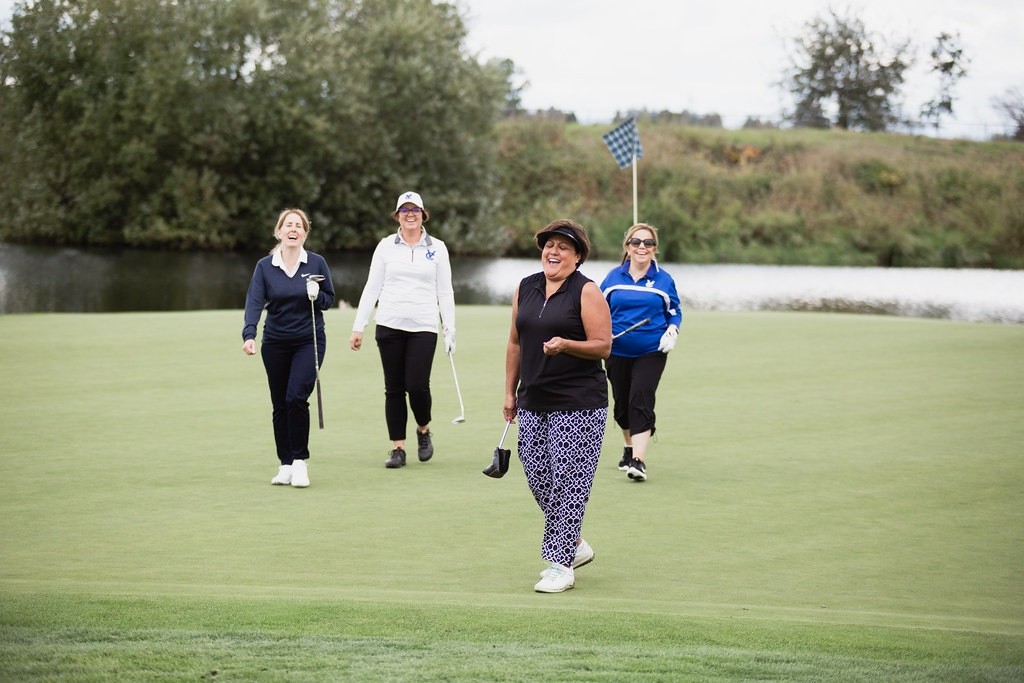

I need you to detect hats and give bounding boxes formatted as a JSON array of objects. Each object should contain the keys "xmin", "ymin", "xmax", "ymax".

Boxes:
[
  {"xmin": 391, "ymin": 191, "xmax": 429, "ymax": 223},
  {"xmin": 536, "ymin": 227, "xmax": 585, "ymax": 257}
]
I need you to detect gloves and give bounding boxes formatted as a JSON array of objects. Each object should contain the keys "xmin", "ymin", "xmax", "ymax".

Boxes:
[
  {"xmin": 444, "ymin": 333, "xmax": 458, "ymax": 357},
  {"xmin": 306, "ymin": 278, "xmax": 319, "ymax": 301},
  {"xmin": 657, "ymin": 328, "xmax": 677, "ymax": 353}
]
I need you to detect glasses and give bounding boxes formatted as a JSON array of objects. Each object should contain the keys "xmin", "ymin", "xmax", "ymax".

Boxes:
[
  {"xmin": 626, "ymin": 238, "xmax": 656, "ymax": 248},
  {"xmin": 399, "ymin": 207, "xmax": 422, "ymax": 216}
]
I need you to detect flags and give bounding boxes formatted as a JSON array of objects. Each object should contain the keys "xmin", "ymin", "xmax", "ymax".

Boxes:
[{"xmin": 602, "ymin": 118, "xmax": 643, "ymax": 169}]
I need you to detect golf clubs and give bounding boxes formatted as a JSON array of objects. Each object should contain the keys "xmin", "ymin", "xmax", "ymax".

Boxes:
[
  {"xmin": 439, "ymin": 312, "xmax": 466, "ymax": 425},
  {"xmin": 309, "ymin": 275, "xmax": 325, "ymax": 429},
  {"xmin": 482, "ymin": 398, "xmax": 518, "ymax": 479},
  {"xmin": 612, "ymin": 318, "xmax": 651, "ymax": 340}
]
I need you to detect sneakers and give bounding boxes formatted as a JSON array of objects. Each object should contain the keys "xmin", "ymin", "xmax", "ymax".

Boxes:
[
  {"xmin": 271, "ymin": 465, "xmax": 291, "ymax": 485},
  {"xmin": 618, "ymin": 447, "xmax": 633, "ymax": 470},
  {"xmin": 540, "ymin": 539, "xmax": 595, "ymax": 579},
  {"xmin": 384, "ymin": 447, "xmax": 407, "ymax": 468},
  {"xmin": 626, "ymin": 457, "xmax": 647, "ymax": 481},
  {"xmin": 534, "ymin": 562, "xmax": 575, "ymax": 592},
  {"xmin": 416, "ymin": 426, "xmax": 434, "ymax": 462},
  {"xmin": 290, "ymin": 459, "xmax": 309, "ymax": 487}
]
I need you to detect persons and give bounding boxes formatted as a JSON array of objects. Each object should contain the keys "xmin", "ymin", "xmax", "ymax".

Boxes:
[
  {"xmin": 600, "ymin": 222, "xmax": 681, "ymax": 481},
  {"xmin": 503, "ymin": 218, "xmax": 613, "ymax": 593},
  {"xmin": 242, "ymin": 209, "xmax": 335, "ymax": 487},
  {"xmin": 349, "ymin": 191, "xmax": 456, "ymax": 468}
]
